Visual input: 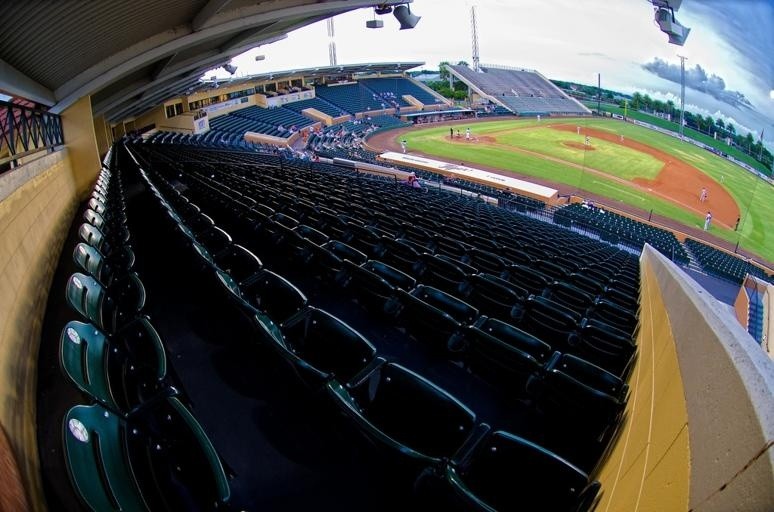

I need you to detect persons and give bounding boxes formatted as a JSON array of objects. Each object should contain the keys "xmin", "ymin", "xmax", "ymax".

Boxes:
[
  {"xmin": 401, "ymin": 141, "xmax": 407, "ymax": 153},
  {"xmin": 704, "ymin": 212, "xmax": 712, "ymax": 231},
  {"xmin": 503, "ymin": 187, "xmax": 517, "ymax": 198},
  {"xmin": 451, "ymin": 127, "xmax": 470, "ymax": 142},
  {"xmin": 734, "ymin": 215, "xmax": 740, "ymax": 231},
  {"xmin": 585, "ymin": 136, "xmax": 591, "ymax": 145},
  {"xmin": 620, "ymin": 134, "xmax": 625, "ymax": 143},
  {"xmin": 582, "ymin": 198, "xmax": 605, "ymax": 214},
  {"xmin": 373, "ymin": 92, "xmax": 400, "ymax": 113},
  {"xmin": 407, "ymin": 172, "xmax": 420, "ymax": 187},
  {"xmin": 277, "ymin": 120, "xmax": 374, "ymax": 162},
  {"xmin": 577, "ymin": 126, "xmax": 580, "ymax": 134}
]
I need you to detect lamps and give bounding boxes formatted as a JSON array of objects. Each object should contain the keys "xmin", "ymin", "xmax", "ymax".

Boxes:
[
  {"xmin": 650, "ymin": 0, "xmax": 692, "ymax": 45},
  {"xmin": 222, "ymin": 63, "xmax": 239, "ymax": 75},
  {"xmin": 392, "ymin": 0, "xmax": 422, "ymax": 30}
]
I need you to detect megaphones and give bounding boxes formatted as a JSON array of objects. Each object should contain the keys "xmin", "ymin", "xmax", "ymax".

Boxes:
[
  {"xmin": 214, "ymin": 84, "xmax": 220, "ymax": 89},
  {"xmin": 393, "ymin": 6, "xmax": 421, "ymax": 30},
  {"xmin": 225, "ymin": 65, "xmax": 237, "ymax": 74},
  {"xmin": 655, "ymin": 9, "xmax": 691, "ymax": 46}
]
